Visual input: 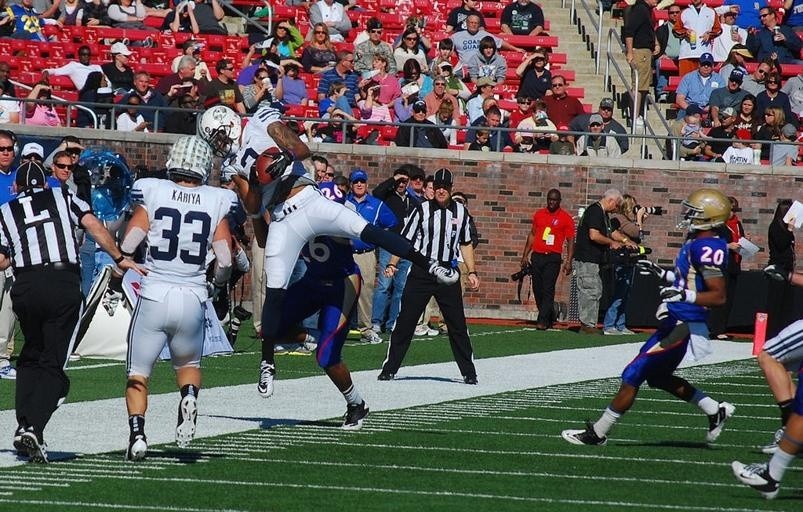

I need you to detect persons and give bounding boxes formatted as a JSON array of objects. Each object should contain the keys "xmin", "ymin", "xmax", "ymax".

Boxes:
[
  {"xmin": 1, "ymin": 129, "xmax": 135, "ymax": 382},
  {"xmin": 754, "ymin": 317, "xmax": 803, "ymax": 454},
  {"xmin": 194, "ymin": 104, "xmax": 464, "ymax": 399},
  {"xmin": 729, "ymin": 363, "xmax": 802, "ymax": 501},
  {"xmin": 0, "ymin": 159, "xmax": 149, "ymax": 467},
  {"xmin": 520, "ymin": 189, "xmax": 575, "ymax": 330},
  {"xmin": 559, "ymin": 185, "xmax": 739, "ymax": 448},
  {"xmin": 1, "ymin": 1, "xmax": 802, "ymax": 165},
  {"xmin": 312, "ymin": 155, "xmax": 480, "ymax": 384},
  {"xmin": 118, "ymin": 136, "xmax": 239, "ymax": 462},
  {"xmin": 276, "ymin": 180, "xmax": 370, "ymax": 432}
]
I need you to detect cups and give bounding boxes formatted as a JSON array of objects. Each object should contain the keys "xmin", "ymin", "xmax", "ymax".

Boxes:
[
  {"xmin": 689, "ymin": 32, "xmax": 696, "ymax": 49},
  {"xmin": 732, "ymin": 26, "xmax": 738, "ymax": 39},
  {"xmin": 773, "ymin": 26, "xmax": 781, "ymax": 42},
  {"xmin": 260, "ymin": 77, "xmax": 273, "ymax": 93}
]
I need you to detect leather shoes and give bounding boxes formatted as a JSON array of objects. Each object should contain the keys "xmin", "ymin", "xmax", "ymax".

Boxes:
[
  {"xmin": 709, "ymin": 332, "xmax": 733, "ymax": 340},
  {"xmin": 536, "ymin": 321, "xmax": 544, "ymax": 329}
]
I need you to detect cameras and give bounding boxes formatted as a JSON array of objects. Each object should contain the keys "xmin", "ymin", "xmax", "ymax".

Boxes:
[
  {"xmin": 633, "ymin": 204, "xmax": 662, "ymax": 216},
  {"xmin": 512, "ymin": 263, "xmax": 532, "ymax": 281},
  {"xmin": 701, "ymin": 119, "xmax": 712, "ymax": 128}
]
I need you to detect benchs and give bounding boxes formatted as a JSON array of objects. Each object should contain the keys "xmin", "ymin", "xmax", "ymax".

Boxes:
[{"xmin": 0, "ymin": 0, "xmax": 802, "ymax": 171}]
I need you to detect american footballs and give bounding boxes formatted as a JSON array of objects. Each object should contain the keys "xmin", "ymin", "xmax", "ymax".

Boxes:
[{"xmin": 255, "ymin": 147, "xmax": 281, "ymax": 186}]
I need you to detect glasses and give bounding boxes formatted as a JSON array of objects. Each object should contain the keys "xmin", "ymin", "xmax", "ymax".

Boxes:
[
  {"xmin": 227, "ymin": 67, "xmax": 234, "ymax": 71},
  {"xmin": 759, "ymin": 14, "xmax": 767, "ymax": 19},
  {"xmin": 55, "ymin": 164, "xmax": 73, "ymax": 170},
  {"xmin": 0, "ymin": 146, "xmax": 13, "ymax": 152},
  {"xmin": 372, "ymin": 30, "xmax": 382, "ymax": 34}
]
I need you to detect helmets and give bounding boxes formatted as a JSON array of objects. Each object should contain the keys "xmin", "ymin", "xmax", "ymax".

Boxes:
[
  {"xmin": 166, "ymin": 105, "xmax": 242, "ymax": 185},
  {"xmin": 681, "ymin": 189, "xmax": 731, "ymax": 230}
]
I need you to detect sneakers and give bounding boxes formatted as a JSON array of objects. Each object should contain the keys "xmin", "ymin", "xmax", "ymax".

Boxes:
[
  {"xmin": 581, "ymin": 328, "xmax": 635, "ymax": 335},
  {"xmin": 124, "ymin": 394, "xmax": 197, "ymax": 461},
  {"xmin": 562, "ymin": 429, "xmax": 608, "ymax": 446},
  {"xmin": 378, "ymin": 372, "xmax": 394, "ymax": 380},
  {"xmin": 707, "ymin": 400, "xmax": 736, "ymax": 443},
  {"xmin": 342, "ymin": 400, "xmax": 368, "ymax": 431},
  {"xmin": 0, "ymin": 366, "xmax": 18, "ymax": 380},
  {"xmin": 762, "ymin": 425, "xmax": 785, "ymax": 454},
  {"xmin": 428, "ymin": 261, "xmax": 459, "ymax": 286},
  {"xmin": 731, "ymin": 460, "xmax": 781, "ymax": 500},
  {"xmin": 257, "ymin": 360, "xmax": 276, "ymax": 398},
  {"xmin": 464, "ymin": 374, "xmax": 478, "ymax": 384},
  {"xmin": 360, "ymin": 325, "xmax": 383, "ymax": 345},
  {"xmin": 273, "ymin": 343, "xmax": 312, "ymax": 355},
  {"xmin": 13, "ymin": 425, "xmax": 50, "ymax": 464},
  {"xmin": 413, "ymin": 325, "xmax": 448, "ymax": 337}
]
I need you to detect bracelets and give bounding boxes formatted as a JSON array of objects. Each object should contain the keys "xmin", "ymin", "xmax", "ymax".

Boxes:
[{"xmin": 112, "ymin": 256, "xmax": 124, "ymax": 263}]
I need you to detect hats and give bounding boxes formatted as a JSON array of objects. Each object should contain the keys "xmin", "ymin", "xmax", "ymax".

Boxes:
[
  {"xmin": 368, "ymin": 22, "xmax": 383, "ymax": 32},
  {"xmin": 110, "ymin": 42, "xmax": 133, "ymax": 56},
  {"xmin": 410, "ymin": 168, "xmax": 453, "ymax": 184},
  {"xmin": 700, "ymin": 49, "xmax": 753, "ymax": 82},
  {"xmin": 686, "ymin": 105, "xmax": 752, "ymax": 140},
  {"xmin": 349, "ymin": 169, "xmax": 368, "ymax": 183},
  {"xmin": 589, "ymin": 98, "xmax": 614, "ymax": 125},
  {"xmin": 476, "ymin": 76, "xmax": 496, "ymax": 87},
  {"xmin": 15, "ymin": 142, "xmax": 46, "ymax": 186}
]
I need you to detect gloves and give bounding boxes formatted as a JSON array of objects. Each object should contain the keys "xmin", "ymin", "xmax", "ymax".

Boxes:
[
  {"xmin": 659, "ymin": 285, "xmax": 697, "ymax": 304},
  {"xmin": 262, "ymin": 151, "xmax": 292, "ymax": 180},
  {"xmin": 762, "ymin": 264, "xmax": 789, "ymax": 282},
  {"xmin": 102, "ymin": 277, "xmax": 127, "ymax": 316},
  {"xmin": 635, "ymin": 260, "xmax": 666, "ymax": 280},
  {"xmin": 249, "ymin": 160, "xmax": 259, "ymax": 187}
]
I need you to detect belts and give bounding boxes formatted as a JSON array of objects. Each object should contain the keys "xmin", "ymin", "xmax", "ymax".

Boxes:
[{"xmin": 17, "ymin": 261, "xmax": 78, "ymax": 270}]
